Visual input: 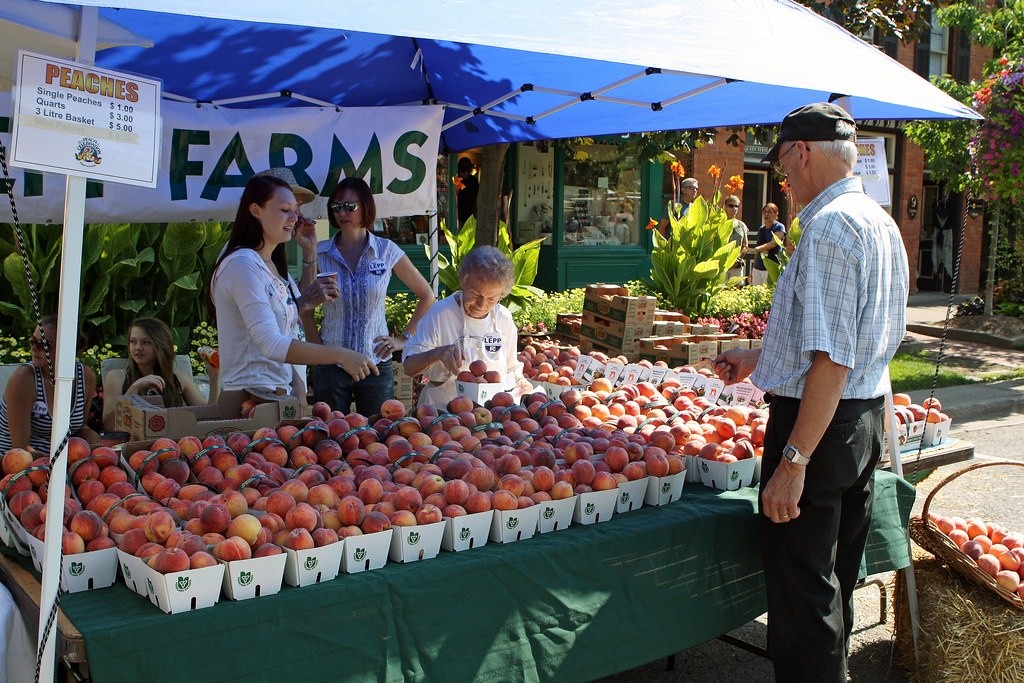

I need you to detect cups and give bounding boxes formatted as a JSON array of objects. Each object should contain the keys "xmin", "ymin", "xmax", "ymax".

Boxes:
[
  {"xmin": 99, "ymin": 431, "xmax": 131, "ymax": 447},
  {"xmin": 316, "ymin": 272, "xmax": 339, "ymax": 298}
]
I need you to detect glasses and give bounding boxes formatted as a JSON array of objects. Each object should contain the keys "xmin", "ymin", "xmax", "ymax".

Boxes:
[
  {"xmin": 726, "ymin": 203, "xmax": 739, "ymax": 208},
  {"xmin": 684, "ymin": 186, "xmax": 697, "ymax": 190},
  {"xmin": 329, "ymin": 201, "xmax": 363, "ymax": 213},
  {"xmin": 771, "ymin": 141, "xmax": 811, "ymax": 177},
  {"xmin": 29, "ymin": 335, "xmax": 53, "ymax": 349}
]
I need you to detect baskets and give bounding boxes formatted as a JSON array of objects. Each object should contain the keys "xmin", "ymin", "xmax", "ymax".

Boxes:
[{"xmin": 909, "ymin": 461, "xmax": 1024, "ymax": 610}]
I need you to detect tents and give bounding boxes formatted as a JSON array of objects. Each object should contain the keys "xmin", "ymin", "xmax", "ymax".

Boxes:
[{"xmin": 0, "ymin": 0, "xmax": 986, "ymax": 683}]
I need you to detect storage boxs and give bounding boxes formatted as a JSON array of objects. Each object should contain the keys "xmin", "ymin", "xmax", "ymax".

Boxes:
[{"xmin": 0, "ymin": 284, "xmax": 953, "ymax": 614}]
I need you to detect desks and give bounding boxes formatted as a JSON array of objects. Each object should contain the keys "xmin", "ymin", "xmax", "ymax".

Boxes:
[{"xmin": 0, "ymin": 436, "xmax": 976, "ymax": 683}]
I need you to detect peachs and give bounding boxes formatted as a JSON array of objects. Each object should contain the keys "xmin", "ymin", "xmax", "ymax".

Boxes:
[
  {"xmin": 457, "ymin": 359, "xmax": 502, "ymax": 383},
  {"xmin": 0, "ymin": 398, "xmax": 689, "ymax": 573},
  {"xmin": 928, "ymin": 512, "xmax": 1024, "ymax": 601},
  {"xmin": 599, "ymin": 293, "xmax": 619, "ymax": 300},
  {"xmin": 518, "ymin": 334, "xmax": 767, "ymax": 464},
  {"xmin": 892, "ymin": 392, "xmax": 949, "ymax": 429}
]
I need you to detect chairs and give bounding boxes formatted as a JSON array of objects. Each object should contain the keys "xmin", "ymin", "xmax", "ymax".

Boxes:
[
  {"xmin": 100, "ymin": 354, "xmax": 193, "ymax": 382},
  {"xmin": 0, "ymin": 364, "xmax": 20, "ymax": 394}
]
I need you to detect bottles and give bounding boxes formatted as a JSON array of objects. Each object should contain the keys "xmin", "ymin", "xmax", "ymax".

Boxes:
[{"xmin": 197, "ymin": 346, "xmax": 219, "ymax": 369}]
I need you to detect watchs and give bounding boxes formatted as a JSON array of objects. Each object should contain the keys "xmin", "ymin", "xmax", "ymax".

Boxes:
[{"xmin": 783, "ymin": 444, "xmax": 810, "ymax": 467}]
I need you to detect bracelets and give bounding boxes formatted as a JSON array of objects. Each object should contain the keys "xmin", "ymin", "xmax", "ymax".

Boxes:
[{"xmin": 401, "ymin": 331, "xmax": 412, "ymax": 341}]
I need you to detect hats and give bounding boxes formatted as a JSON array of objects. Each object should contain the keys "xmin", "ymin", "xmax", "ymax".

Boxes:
[{"xmin": 760, "ymin": 102, "xmax": 857, "ymax": 163}]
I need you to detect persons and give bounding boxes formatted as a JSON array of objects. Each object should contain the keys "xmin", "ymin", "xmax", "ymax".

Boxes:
[
  {"xmin": 103, "ymin": 316, "xmax": 221, "ymax": 437},
  {"xmin": 303, "ymin": 176, "xmax": 434, "ymax": 420},
  {"xmin": 748, "ymin": 203, "xmax": 786, "ymax": 286},
  {"xmin": 458, "ymin": 157, "xmax": 480, "ymax": 234},
  {"xmin": 1, "ymin": 315, "xmax": 101, "ymax": 462},
  {"xmin": 721, "ymin": 195, "xmax": 749, "ymax": 291},
  {"xmin": 402, "ymin": 246, "xmax": 533, "ymax": 414},
  {"xmin": 711, "ymin": 104, "xmax": 910, "ymax": 682},
  {"xmin": 661, "ymin": 177, "xmax": 699, "ymax": 245},
  {"xmin": 207, "ymin": 166, "xmax": 381, "ymax": 418}
]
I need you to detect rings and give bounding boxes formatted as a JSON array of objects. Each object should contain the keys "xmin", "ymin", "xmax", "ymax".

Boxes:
[
  {"xmin": 781, "ymin": 514, "xmax": 788, "ymax": 519},
  {"xmin": 386, "ymin": 345, "xmax": 391, "ymax": 349},
  {"xmin": 772, "ymin": 516, "xmax": 778, "ymax": 523}
]
